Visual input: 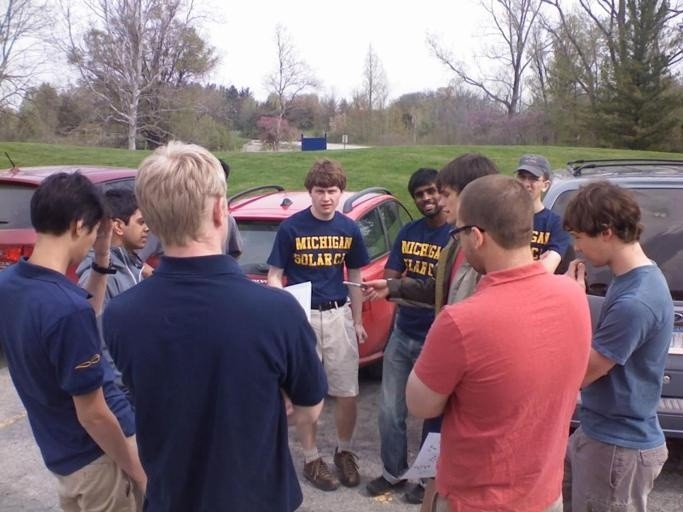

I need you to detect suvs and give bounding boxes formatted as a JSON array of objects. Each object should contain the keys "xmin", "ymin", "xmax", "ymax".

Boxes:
[
  {"xmin": 530, "ymin": 155, "xmax": 682, "ymax": 440},
  {"xmin": 141, "ymin": 183, "xmax": 417, "ymax": 384},
  {"xmin": 1, "ymin": 152, "xmax": 158, "ymax": 326}
]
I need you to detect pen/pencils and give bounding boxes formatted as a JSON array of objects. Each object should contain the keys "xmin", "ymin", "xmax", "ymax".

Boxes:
[{"xmin": 343, "ymin": 281, "xmax": 368, "ymax": 288}]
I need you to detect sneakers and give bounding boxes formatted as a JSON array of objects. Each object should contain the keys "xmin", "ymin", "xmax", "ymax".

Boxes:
[
  {"xmin": 303, "ymin": 456, "xmax": 340, "ymax": 490},
  {"xmin": 405, "ymin": 483, "xmax": 425, "ymax": 504},
  {"xmin": 366, "ymin": 475, "xmax": 408, "ymax": 496},
  {"xmin": 334, "ymin": 446, "xmax": 360, "ymax": 487}
]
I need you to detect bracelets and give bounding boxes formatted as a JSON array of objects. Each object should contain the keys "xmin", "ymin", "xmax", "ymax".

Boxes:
[{"xmin": 91, "ymin": 261, "xmax": 117, "ymax": 276}]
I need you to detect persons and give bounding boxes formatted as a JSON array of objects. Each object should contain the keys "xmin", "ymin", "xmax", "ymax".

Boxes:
[
  {"xmin": 75, "ymin": 186, "xmax": 154, "ymax": 399},
  {"xmin": 562, "ymin": 179, "xmax": 674, "ymax": 512},
  {"xmin": 265, "ymin": 158, "xmax": 369, "ymax": 490},
  {"xmin": 513, "ymin": 154, "xmax": 571, "ymax": 273},
  {"xmin": 367, "ymin": 165, "xmax": 457, "ymax": 505},
  {"xmin": 402, "ymin": 172, "xmax": 592, "ymax": 512},
  {"xmin": 217, "ymin": 158, "xmax": 243, "ymax": 259},
  {"xmin": 100, "ymin": 142, "xmax": 330, "ymax": 512},
  {"xmin": 0, "ymin": 167, "xmax": 146, "ymax": 512},
  {"xmin": 357, "ymin": 151, "xmax": 498, "ymax": 322}
]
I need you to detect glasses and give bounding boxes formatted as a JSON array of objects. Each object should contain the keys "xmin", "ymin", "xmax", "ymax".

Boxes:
[{"xmin": 448, "ymin": 225, "xmax": 485, "ymax": 241}]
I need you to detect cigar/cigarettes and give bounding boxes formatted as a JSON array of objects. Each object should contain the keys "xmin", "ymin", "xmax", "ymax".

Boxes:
[{"xmin": 342, "ymin": 280, "xmax": 364, "ymax": 290}]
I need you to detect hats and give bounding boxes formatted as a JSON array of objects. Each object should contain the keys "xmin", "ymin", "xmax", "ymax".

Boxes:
[{"xmin": 513, "ymin": 154, "xmax": 551, "ymax": 180}]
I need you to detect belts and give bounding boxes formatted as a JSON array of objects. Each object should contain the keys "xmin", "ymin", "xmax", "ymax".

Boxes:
[{"xmin": 311, "ymin": 296, "xmax": 347, "ymax": 311}]
